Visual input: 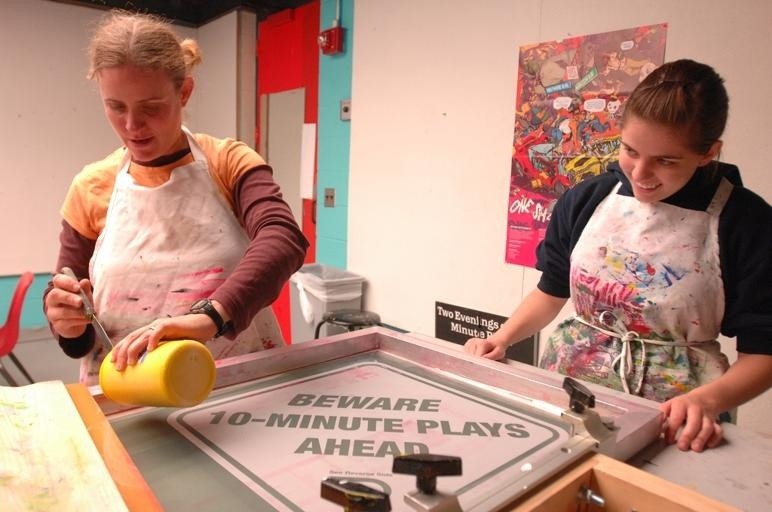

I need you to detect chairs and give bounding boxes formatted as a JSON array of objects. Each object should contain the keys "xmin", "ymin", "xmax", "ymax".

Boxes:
[{"xmin": 0, "ymin": 271, "xmax": 34, "ymax": 387}]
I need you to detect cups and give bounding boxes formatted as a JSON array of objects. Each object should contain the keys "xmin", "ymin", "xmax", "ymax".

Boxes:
[{"xmin": 98, "ymin": 338, "xmax": 218, "ymax": 408}]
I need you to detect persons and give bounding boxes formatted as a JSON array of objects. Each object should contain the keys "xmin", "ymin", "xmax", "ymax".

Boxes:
[
  {"xmin": 44, "ymin": 13, "xmax": 310, "ymax": 387},
  {"xmin": 464, "ymin": 60, "xmax": 772, "ymax": 452}
]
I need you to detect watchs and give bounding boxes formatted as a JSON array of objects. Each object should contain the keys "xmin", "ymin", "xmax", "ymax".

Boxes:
[{"xmin": 184, "ymin": 298, "xmax": 228, "ymax": 338}]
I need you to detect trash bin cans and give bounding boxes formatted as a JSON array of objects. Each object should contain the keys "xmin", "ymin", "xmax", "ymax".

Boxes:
[{"xmin": 290, "ymin": 265, "xmax": 365, "ymax": 341}]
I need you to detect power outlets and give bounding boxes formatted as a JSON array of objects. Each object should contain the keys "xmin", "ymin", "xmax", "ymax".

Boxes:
[{"xmin": 324, "ymin": 189, "xmax": 335, "ymax": 207}]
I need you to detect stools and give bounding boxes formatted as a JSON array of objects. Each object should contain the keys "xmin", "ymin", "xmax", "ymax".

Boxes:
[{"xmin": 315, "ymin": 309, "xmax": 383, "ymax": 339}]
